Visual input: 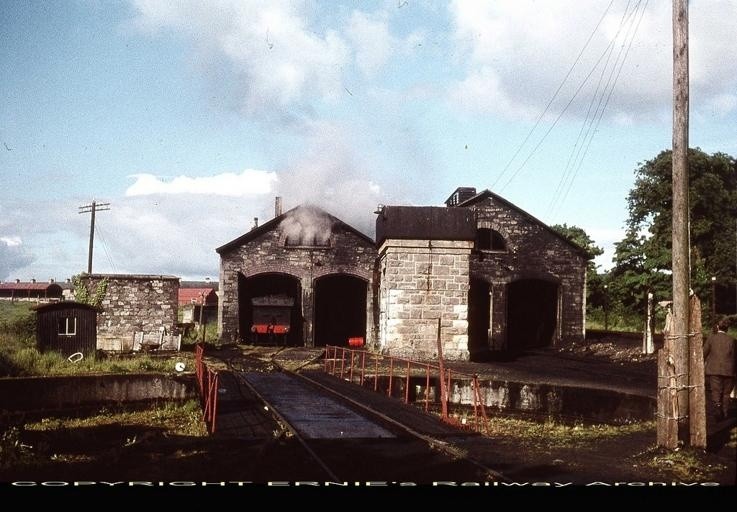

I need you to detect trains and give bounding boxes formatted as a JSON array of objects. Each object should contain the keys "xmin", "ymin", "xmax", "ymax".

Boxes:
[{"xmin": 250, "ymin": 287, "xmax": 292, "ymax": 346}]
